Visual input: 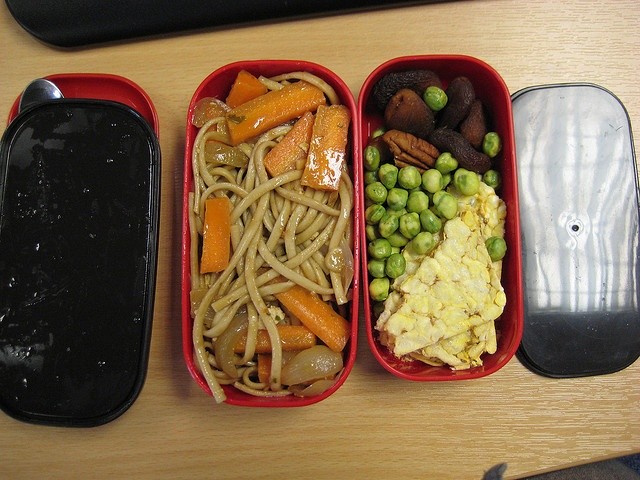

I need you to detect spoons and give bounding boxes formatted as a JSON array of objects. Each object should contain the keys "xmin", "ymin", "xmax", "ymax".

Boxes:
[{"xmin": 18, "ymin": 77, "xmax": 65, "ymax": 114}]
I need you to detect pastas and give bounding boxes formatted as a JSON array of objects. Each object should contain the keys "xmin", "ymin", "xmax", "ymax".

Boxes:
[{"xmin": 188, "ymin": 72, "xmax": 354, "ymax": 403}]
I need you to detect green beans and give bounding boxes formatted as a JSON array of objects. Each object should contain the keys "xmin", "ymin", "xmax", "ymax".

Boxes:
[{"xmin": 365, "ymin": 86, "xmax": 507, "ymax": 302}]
